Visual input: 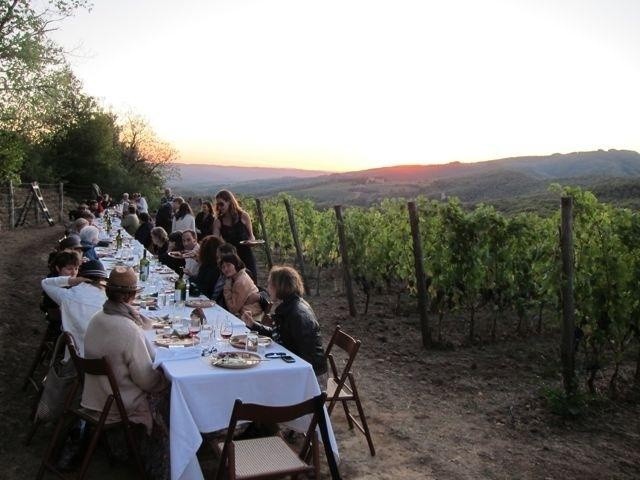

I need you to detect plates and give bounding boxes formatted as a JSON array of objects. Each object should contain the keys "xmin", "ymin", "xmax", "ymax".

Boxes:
[
  {"xmin": 228, "ymin": 334, "xmax": 274, "ymax": 349},
  {"xmin": 211, "ymin": 352, "xmax": 263, "ymax": 370}
]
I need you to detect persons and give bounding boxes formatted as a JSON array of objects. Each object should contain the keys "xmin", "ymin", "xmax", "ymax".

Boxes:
[{"xmin": 39, "ymin": 189, "xmax": 329, "ymax": 443}]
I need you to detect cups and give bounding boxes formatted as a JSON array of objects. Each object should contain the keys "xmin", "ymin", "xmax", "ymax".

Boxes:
[
  {"xmin": 247, "ymin": 330, "xmax": 258, "ymax": 357},
  {"xmin": 95, "ymin": 201, "xmax": 233, "ymax": 361}
]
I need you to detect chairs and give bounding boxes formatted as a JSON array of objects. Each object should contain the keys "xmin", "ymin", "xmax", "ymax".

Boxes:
[
  {"xmin": 22, "ymin": 308, "xmax": 60, "ymax": 389},
  {"xmin": 36, "ymin": 331, "xmax": 145, "ymax": 480},
  {"xmin": 324, "ymin": 325, "xmax": 375, "ymax": 456}
]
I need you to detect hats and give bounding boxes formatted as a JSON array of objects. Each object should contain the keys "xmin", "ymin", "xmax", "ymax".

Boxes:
[
  {"xmin": 99, "ymin": 266, "xmax": 145, "ymax": 293},
  {"xmin": 77, "ymin": 260, "xmax": 105, "ymax": 279}
]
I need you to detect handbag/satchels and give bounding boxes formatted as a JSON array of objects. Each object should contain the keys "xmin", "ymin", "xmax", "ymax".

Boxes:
[{"xmin": 37, "ymin": 331, "xmax": 82, "ymax": 420}]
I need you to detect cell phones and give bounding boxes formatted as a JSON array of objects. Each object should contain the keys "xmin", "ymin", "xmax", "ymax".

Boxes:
[{"xmin": 279, "ymin": 352, "xmax": 295, "ymax": 363}]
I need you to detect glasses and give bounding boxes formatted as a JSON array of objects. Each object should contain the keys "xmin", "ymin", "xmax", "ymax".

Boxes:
[
  {"xmin": 201, "ymin": 347, "xmax": 217, "ymax": 357},
  {"xmin": 216, "ymin": 202, "xmax": 224, "ymax": 206}
]
